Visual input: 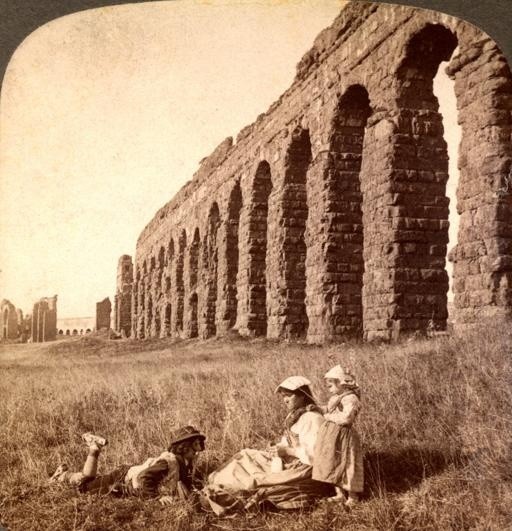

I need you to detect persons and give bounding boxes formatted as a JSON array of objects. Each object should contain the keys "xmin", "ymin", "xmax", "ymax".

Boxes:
[
  {"xmin": 47, "ymin": 425, "xmax": 206, "ymax": 507},
  {"xmin": 202, "ymin": 375, "xmax": 328, "ymax": 511},
  {"xmin": 306, "ymin": 362, "xmax": 368, "ymax": 509}
]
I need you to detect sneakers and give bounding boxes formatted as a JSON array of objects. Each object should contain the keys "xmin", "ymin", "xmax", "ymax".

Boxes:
[
  {"xmin": 49, "ymin": 463, "xmax": 68, "ymax": 485},
  {"xmin": 82, "ymin": 434, "xmax": 108, "ymax": 451}
]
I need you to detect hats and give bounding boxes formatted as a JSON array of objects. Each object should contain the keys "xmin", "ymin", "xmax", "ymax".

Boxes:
[{"xmin": 168, "ymin": 425, "xmax": 206, "ymax": 447}]
